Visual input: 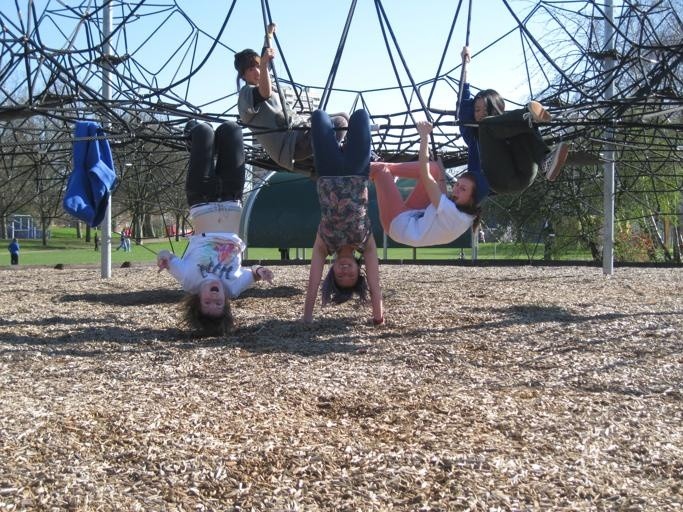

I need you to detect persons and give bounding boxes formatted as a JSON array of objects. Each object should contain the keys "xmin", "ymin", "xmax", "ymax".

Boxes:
[
  {"xmin": 116, "ymin": 228, "xmax": 127, "ymax": 253},
  {"xmin": 124, "ymin": 226, "xmax": 131, "ymax": 252},
  {"xmin": 93, "ymin": 231, "xmax": 100, "ymax": 252},
  {"xmin": 454, "ymin": 44, "xmax": 572, "ymax": 199},
  {"xmin": 153, "ymin": 114, "xmax": 277, "ymax": 338},
  {"xmin": 232, "ymin": 20, "xmax": 351, "ymax": 184},
  {"xmin": 299, "ymin": 109, "xmax": 385, "ymax": 327},
  {"xmin": 365, "ymin": 121, "xmax": 479, "ymax": 248},
  {"xmin": 7, "ymin": 237, "xmax": 19, "ymax": 265},
  {"xmin": 542, "ymin": 221, "xmax": 555, "ymax": 261}
]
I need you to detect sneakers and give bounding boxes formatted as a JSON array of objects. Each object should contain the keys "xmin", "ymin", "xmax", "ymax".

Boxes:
[
  {"xmin": 527, "ymin": 100, "xmax": 553, "ymax": 124},
  {"xmin": 183, "ymin": 119, "xmax": 214, "ymax": 155},
  {"xmin": 539, "ymin": 141, "xmax": 570, "ymax": 184}
]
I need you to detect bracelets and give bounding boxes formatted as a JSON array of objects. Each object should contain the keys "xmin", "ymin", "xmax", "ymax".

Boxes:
[{"xmin": 263, "ymin": 34, "xmax": 271, "ymax": 39}]
